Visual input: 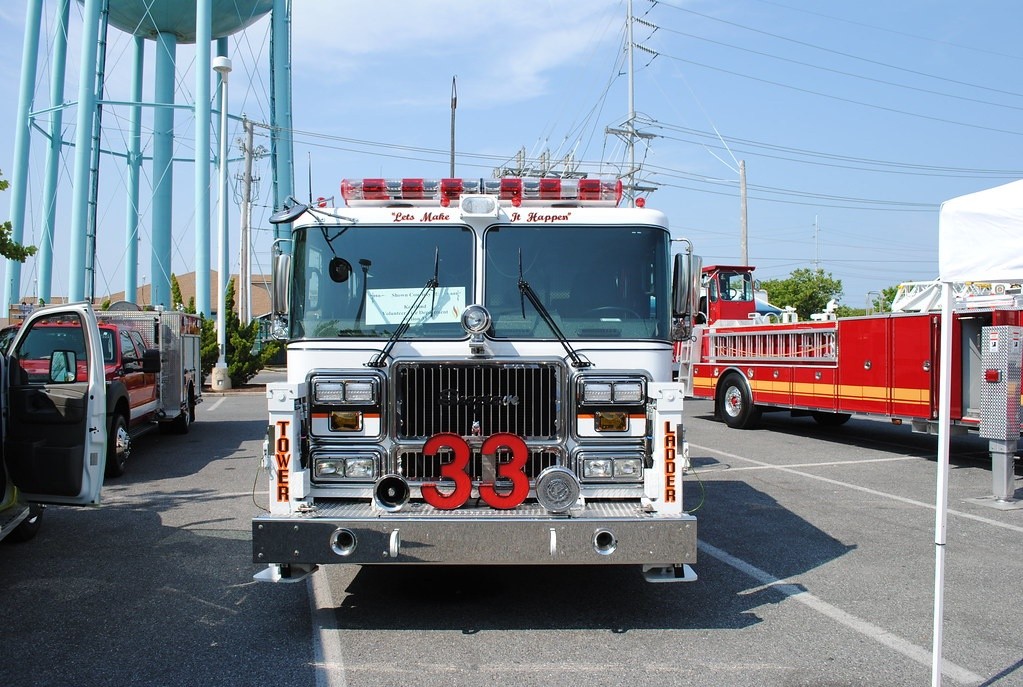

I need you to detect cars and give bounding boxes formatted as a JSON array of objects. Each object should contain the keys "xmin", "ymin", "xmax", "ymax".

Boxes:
[{"xmin": 0, "ymin": 299, "xmax": 108, "ymax": 544}]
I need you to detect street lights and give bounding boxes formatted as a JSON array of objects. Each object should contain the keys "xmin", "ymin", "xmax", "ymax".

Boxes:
[{"xmin": 212, "ymin": 56, "xmax": 232, "ymax": 391}]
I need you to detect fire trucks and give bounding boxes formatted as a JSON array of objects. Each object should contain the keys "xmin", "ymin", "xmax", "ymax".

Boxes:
[
  {"xmin": 669, "ymin": 264, "xmax": 1023, "ymax": 511},
  {"xmin": 0, "ymin": 310, "xmax": 203, "ymax": 479},
  {"xmin": 252, "ymin": 178, "xmax": 703, "ymax": 583}
]
